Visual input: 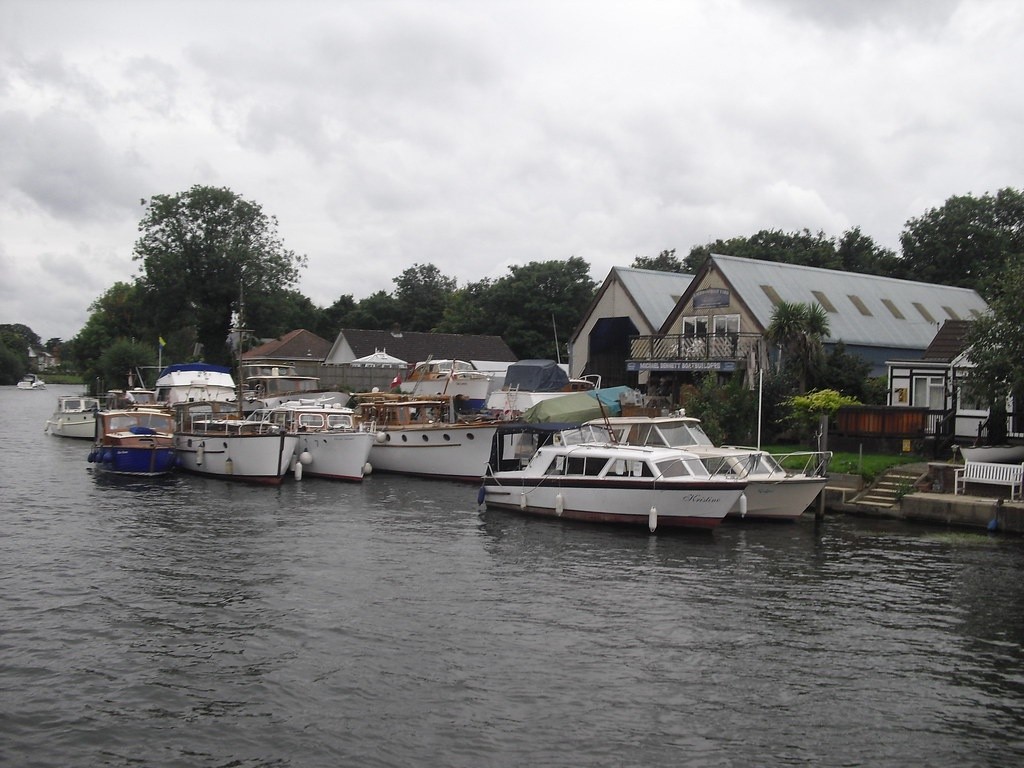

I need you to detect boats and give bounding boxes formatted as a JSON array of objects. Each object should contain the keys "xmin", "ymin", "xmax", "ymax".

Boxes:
[
  {"xmin": 45, "ymin": 278, "xmax": 591, "ymax": 487},
  {"xmin": 17, "ymin": 373, "xmax": 48, "ymax": 390},
  {"xmin": 531, "ymin": 400, "xmax": 835, "ymax": 524},
  {"xmin": 475, "ymin": 390, "xmax": 765, "ymax": 530}
]
